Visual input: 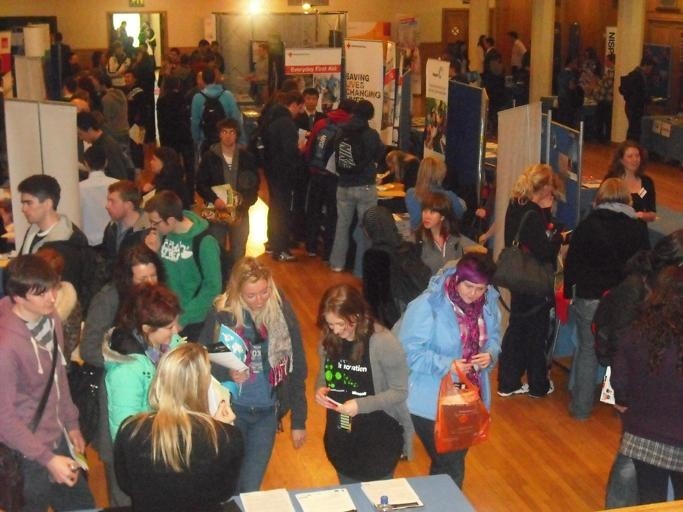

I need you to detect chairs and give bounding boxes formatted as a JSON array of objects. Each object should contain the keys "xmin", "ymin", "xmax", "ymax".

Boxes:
[
  {"xmin": 496, "ymin": 381, "xmax": 554, "ymax": 399},
  {"xmin": 264, "ymin": 239, "xmax": 344, "ymax": 272}
]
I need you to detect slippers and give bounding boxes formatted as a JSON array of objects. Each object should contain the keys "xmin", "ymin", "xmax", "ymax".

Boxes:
[
  {"xmin": 334, "ymin": 123, "xmax": 377, "ymax": 176},
  {"xmin": 198, "ymin": 88, "xmax": 229, "ymax": 144},
  {"xmin": 308, "ymin": 117, "xmax": 349, "ymax": 177}
]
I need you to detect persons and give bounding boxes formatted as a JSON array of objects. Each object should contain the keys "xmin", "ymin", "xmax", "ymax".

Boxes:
[
  {"xmin": 78, "ymin": 109, "xmax": 259, "ymax": 509},
  {"xmin": 113, "ymin": 341, "xmax": 244, "ymax": 512},
  {"xmin": 260, "ymin": 80, "xmax": 388, "ymax": 275},
  {"xmin": 196, "ymin": 257, "xmax": 306, "ymax": 494},
  {"xmin": 18, "ymin": 175, "xmax": 92, "ymax": 300},
  {"xmin": 360, "ymin": 149, "xmax": 493, "ymax": 330},
  {"xmin": 395, "ymin": 253, "xmax": 505, "ymax": 489},
  {"xmin": 620, "ymin": 261, "xmax": 682, "ymax": 508},
  {"xmin": 452, "ymin": 29, "xmax": 657, "ymax": 142},
  {"xmin": 497, "ymin": 141, "xmax": 655, "ymax": 420},
  {"xmin": 248, "ymin": 44, "xmax": 269, "ymax": 107},
  {"xmin": 590, "ymin": 230, "xmax": 683, "ymax": 512},
  {"xmin": 312, "ymin": 283, "xmax": 410, "ymax": 486},
  {"xmin": 46, "ymin": 21, "xmax": 247, "ymax": 146},
  {"xmin": 0, "ymin": 255, "xmax": 94, "ymax": 510}
]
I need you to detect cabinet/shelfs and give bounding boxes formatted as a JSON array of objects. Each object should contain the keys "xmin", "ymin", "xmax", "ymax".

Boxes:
[{"xmin": 376, "ymin": 495, "xmax": 392, "ymax": 512}]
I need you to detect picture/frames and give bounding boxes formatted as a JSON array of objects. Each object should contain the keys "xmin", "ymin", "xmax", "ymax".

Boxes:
[
  {"xmin": 234, "ymin": 473, "xmax": 477, "ymax": 512},
  {"xmin": 244, "ymin": 106, "xmax": 683, "ymax": 258}
]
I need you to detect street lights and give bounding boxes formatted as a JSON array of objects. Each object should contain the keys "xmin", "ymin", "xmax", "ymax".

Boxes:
[
  {"xmin": 488, "ymin": 210, "xmax": 556, "ymax": 299},
  {"xmin": 65, "ymin": 359, "xmax": 105, "ymax": 436},
  {"xmin": 1, "ymin": 443, "xmax": 25, "ymax": 485}
]
